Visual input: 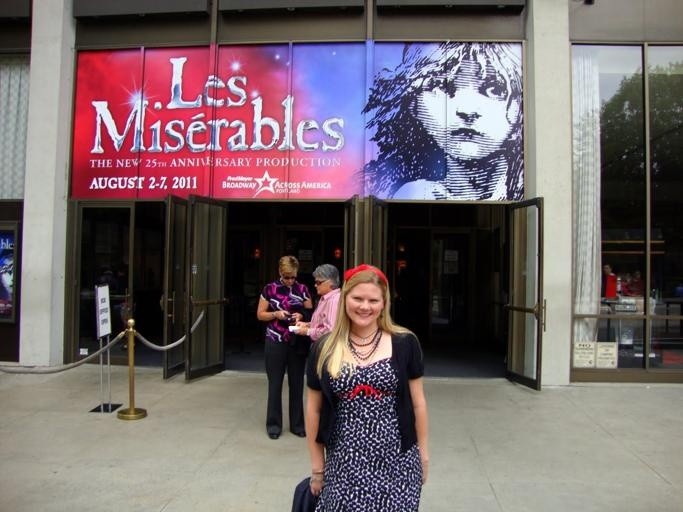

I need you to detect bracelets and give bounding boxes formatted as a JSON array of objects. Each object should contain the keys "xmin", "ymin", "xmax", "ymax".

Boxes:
[{"xmin": 312, "ymin": 470, "xmax": 325, "ymax": 475}]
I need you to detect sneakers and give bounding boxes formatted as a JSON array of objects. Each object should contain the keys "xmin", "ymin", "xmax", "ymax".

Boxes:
[{"xmin": 267, "ymin": 428, "xmax": 307, "ymax": 439}]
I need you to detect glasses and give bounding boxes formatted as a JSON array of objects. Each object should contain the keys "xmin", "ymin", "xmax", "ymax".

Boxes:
[
  {"xmin": 283, "ymin": 276, "xmax": 296, "ymax": 280},
  {"xmin": 315, "ymin": 280, "xmax": 327, "ymax": 285}
]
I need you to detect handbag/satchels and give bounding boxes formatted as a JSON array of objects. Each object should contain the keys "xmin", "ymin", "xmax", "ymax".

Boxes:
[{"xmin": 291, "ymin": 477, "xmax": 316, "ymax": 512}]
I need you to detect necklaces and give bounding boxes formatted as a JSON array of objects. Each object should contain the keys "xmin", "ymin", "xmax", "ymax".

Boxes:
[{"xmin": 344, "ymin": 328, "xmax": 383, "ymax": 367}]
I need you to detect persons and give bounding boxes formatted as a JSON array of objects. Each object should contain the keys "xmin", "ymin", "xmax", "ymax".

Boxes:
[
  {"xmin": 0, "ymin": 247, "xmax": 14, "ymax": 304},
  {"xmin": 255, "ymin": 256, "xmax": 342, "ymax": 438},
  {"xmin": 363, "ymin": 44, "xmax": 522, "ymax": 201},
  {"xmin": 604, "ymin": 264, "xmax": 644, "ymax": 298},
  {"xmin": 291, "ymin": 265, "xmax": 428, "ymax": 512}
]
